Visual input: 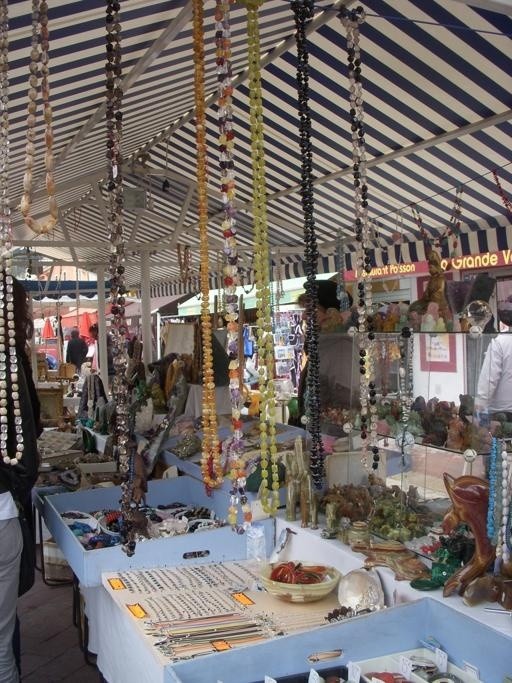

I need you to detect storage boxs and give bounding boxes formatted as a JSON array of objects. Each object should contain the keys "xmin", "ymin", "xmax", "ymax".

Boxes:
[
  {"xmin": 164, "ymin": 420, "xmax": 304, "ymax": 472},
  {"xmin": 184, "ymin": 448, "xmax": 412, "ymax": 508},
  {"xmin": 44, "ymin": 475, "xmax": 275, "ymax": 587},
  {"xmin": 43, "ymin": 542, "xmax": 73, "ymax": 580},
  {"xmin": 32, "ymin": 486, "xmax": 70, "ymax": 517},
  {"xmin": 164, "ymin": 598, "xmax": 511, "ymax": 683}
]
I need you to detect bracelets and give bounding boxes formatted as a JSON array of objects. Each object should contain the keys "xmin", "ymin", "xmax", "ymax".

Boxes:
[{"xmin": 271, "ymin": 560, "xmax": 327, "ymax": 586}]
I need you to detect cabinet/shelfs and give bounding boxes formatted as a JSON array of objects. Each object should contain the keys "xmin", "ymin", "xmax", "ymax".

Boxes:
[{"xmin": 348, "ymin": 332, "xmax": 512, "ymax": 561}]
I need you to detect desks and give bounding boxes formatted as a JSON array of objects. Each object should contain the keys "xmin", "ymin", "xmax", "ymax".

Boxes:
[{"xmin": 275, "ymin": 507, "xmax": 512, "ymax": 641}]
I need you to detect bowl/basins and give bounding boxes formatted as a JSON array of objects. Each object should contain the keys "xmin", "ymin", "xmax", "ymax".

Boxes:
[
  {"xmin": 337, "ymin": 569, "xmax": 380, "ymax": 610},
  {"xmin": 260, "ymin": 566, "xmax": 341, "ymax": 603}
]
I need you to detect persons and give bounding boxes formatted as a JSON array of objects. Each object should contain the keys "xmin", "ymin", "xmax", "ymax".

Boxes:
[
  {"xmin": 0, "ymin": 271, "xmax": 42, "ymax": 682},
  {"xmin": 473, "ymin": 309, "xmax": 512, "ymax": 414},
  {"xmin": 288, "ymin": 280, "xmax": 359, "ymax": 430},
  {"xmin": 66, "ymin": 330, "xmax": 88, "ymax": 372}
]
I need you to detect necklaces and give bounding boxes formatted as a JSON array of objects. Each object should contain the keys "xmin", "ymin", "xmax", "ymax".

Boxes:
[
  {"xmin": 1, "ymin": 2, "xmax": 26, "ymax": 466},
  {"xmin": 410, "ymin": 185, "xmax": 462, "ymax": 244},
  {"xmin": 374, "ymin": 207, "xmax": 403, "ymax": 296},
  {"xmin": 244, "ymin": 3, "xmax": 281, "ymax": 517},
  {"xmin": 485, "ymin": 436, "xmax": 511, "ymax": 578},
  {"xmin": 215, "ymin": 3, "xmax": 244, "ymax": 537},
  {"xmin": 21, "ymin": 1, "xmax": 58, "ymax": 235},
  {"xmin": 344, "ymin": 4, "xmax": 381, "ymax": 473},
  {"xmin": 292, "ymin": 3, "xmax": 326, "ymax": 493},
  {"xmin": 189, "ymin": 0, "xmax": 221, "ymax": 500},
  {"xmin": 102, "ymin": 1, "xmax": 141, "ymax": 556},
  {"xmin": 493, "ymin": 169, "xmax": 512, "ymax": 212}
]
